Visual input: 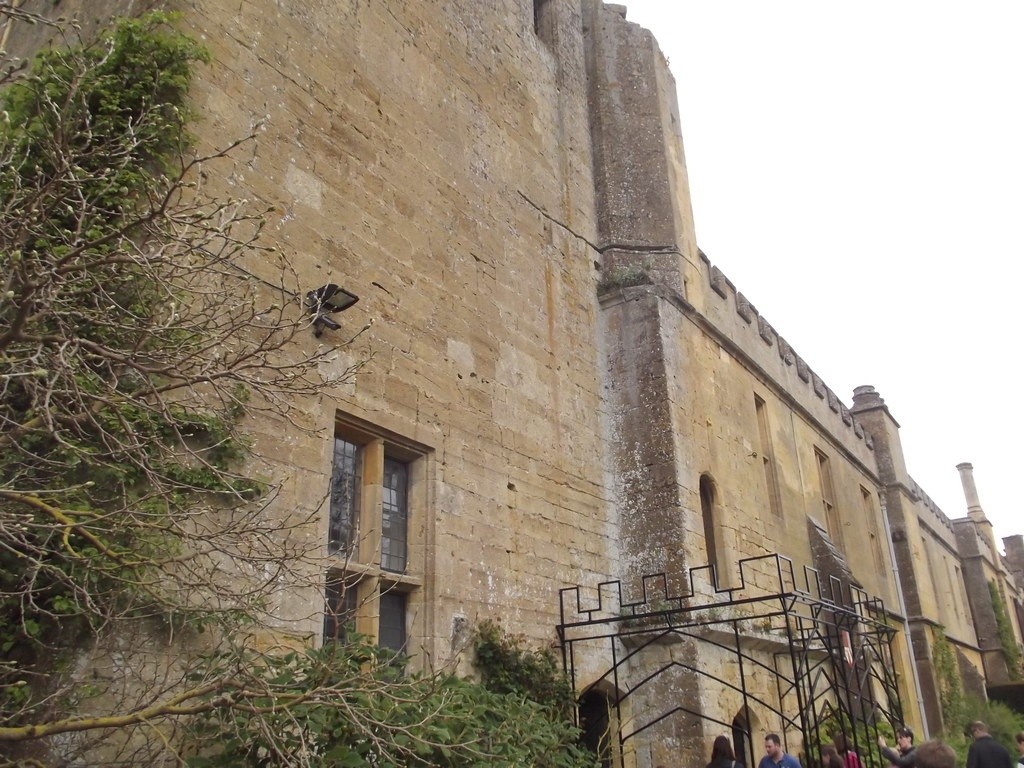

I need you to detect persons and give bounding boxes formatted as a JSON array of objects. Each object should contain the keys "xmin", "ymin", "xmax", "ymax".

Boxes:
[
  {"xmin": 877, "ymin": 727, "xmax": 921, "ymax": 768},
  {"xmin": 1014, "ymin": 731, "xmax": 1024, "ymax": 768},
  {"xmin": 915, "ymin": 740, "xmax": 956, "ymax": 768},
  {"xmin": 820, "ymin": 732, "xmax": 860, "ymax": 768},
  {"xmin": 758, "ymin": 734, "xmax": 802, "ymax": 768},
  {"xmin": 707, "ymin": 736, "xmax": 745, "ymax": 768},
  {"xmin": 964, "ymin": 720, "xmax": 1015, "ymax": 768}
]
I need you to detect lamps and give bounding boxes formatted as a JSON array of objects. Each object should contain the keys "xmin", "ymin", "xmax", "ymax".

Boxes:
[{"xmin": 307, "ymin": 284, "xmax": 360, "ymax": 339}]
